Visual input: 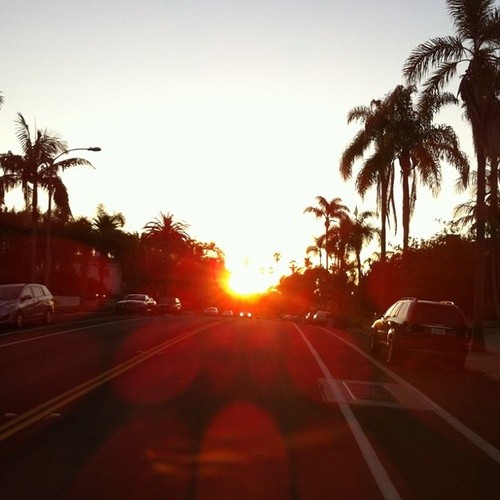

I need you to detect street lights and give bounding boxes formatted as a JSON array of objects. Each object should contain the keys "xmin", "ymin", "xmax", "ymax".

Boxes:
[{"xmin": 44, "ymin": 147, "xmax": 102, "ymax": 290}]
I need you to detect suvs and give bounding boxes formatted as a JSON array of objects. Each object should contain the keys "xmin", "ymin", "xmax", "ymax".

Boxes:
[{"xmin": 367, "ymin": 296, "xmax": 471, "ymax": 372}]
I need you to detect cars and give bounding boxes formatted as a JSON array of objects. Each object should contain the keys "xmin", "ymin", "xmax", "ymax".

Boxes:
[
  {"xmin": 203, "ymin": 307, "xmax": 218, "ymax": 316},
  {"xmin": 222, "ymin": 310, "xmax": 234, "ymax": 317},
  {"xmin": 115, "ymin": 293, "xmax": 157, "ymax": 315},
  {"xmin": 239, "ymin": 309, "xmax": 252, "ymax": 318},
  {"xmin": 157, "ymin": 296, "xmax": 181, "ymax": 314},
  {"xmin": 313, "ymin": 310, "xmax": 336, "ymax": 325},
  {"xmin": 0, "ymin": 284, "xmax": 55, "ymax": 329},
  {"xmin": 304, "ymin": 311, "xmax": 316, "ymax": 323}
]
